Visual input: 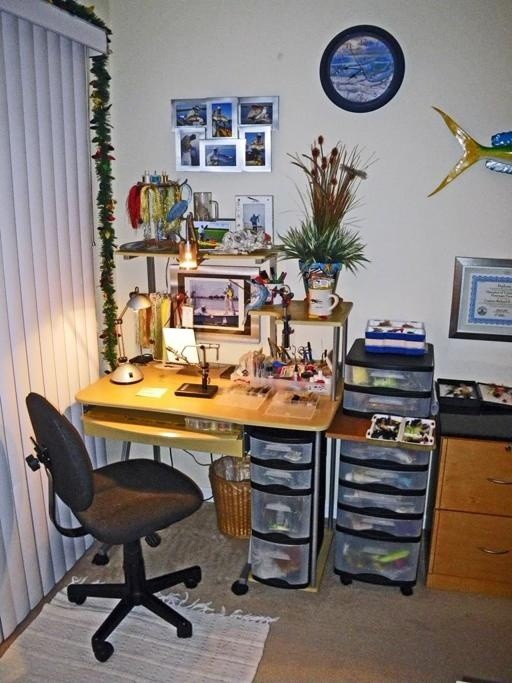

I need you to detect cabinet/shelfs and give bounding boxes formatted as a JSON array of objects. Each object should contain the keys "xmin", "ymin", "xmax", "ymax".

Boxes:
[
  {"xmin": 247, "ymin": 427, "xmax": 324, "ymax": 590},
  {"xmin": 425, "ymin": 435, "xmax": 512, "ymax": 600},
  {"xmin": 76, "ymin": 249, "xmax": 353, "ymax": 595},
  {"xmin": 343, "ymin": 338, "xmax": 434, "ymax": 419},
  {"xmin": 333, "ymin": 439, "xmax": 431, "ymax": 597}
]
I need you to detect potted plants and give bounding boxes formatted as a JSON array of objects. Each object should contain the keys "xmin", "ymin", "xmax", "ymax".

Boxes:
[{"xmin": 262, "ymin": 217, "xmax": 372, "ymax": 308}]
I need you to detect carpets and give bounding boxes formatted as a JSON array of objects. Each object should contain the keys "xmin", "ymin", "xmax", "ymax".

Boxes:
[{"xmin": 1, "ymin": 577, "xmax": 279, "ymax": 683}]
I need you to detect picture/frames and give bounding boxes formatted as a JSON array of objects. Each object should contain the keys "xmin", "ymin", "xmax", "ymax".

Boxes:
[
  {"xmin": 320, "ymin": 24, "xmax": 405, "ymax": 113},
  {"xmin": 170, "ymin": 96, "xmax": 279, "ymax": 173},
  {"xmin": 448, "ymin": 256, "xmax": 512, "ymax": 342},
  {"xmin": 234, "ymin": 195, "xmax": 274, "ymax": 250},
  {"xmin": 177, "ymin": 273, "xmax": 253, "ymax": 335}
]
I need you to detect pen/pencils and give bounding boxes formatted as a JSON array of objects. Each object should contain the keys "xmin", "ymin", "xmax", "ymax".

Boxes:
[
  {"xmin": 268, "ymin": 338, "xmax": 291, "ymax": 364},
  {"xmin": 252, "ymin": 267, "xmax": 287, "ymax": 286},
  {"xmin": 308, "ymin": 342, "xmax": 312, "ymax": 361},
  {"xmin": 303, "ymin": 347, "xmax": 309, "ymax": 361}
]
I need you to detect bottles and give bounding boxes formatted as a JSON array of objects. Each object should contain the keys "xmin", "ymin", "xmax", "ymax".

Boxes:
[{"xmin": 143, "ymin": 169, "xmax": 170, "ymax": 184}]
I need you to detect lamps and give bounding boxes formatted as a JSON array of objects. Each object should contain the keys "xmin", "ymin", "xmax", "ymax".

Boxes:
[{"xmin": 110, "ymin": 286, "xmax": 153, "ymax": 385}]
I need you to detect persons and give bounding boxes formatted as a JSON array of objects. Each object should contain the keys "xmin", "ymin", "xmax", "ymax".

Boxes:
[
  {"xmin": 223, "ymin": 283, "xmax": 236, "ymax": 316},
  {"xmin": 250, "ymin": 214, "xmax": 259, "ymax": 230},
  {"xmin": 180, "ymin": 104, "xmax": 268, "ymax": 166},
  {"xmin": 194, "ymin": 305, "xmax": 211, "ymax": 316},
  {"xmin": 189, "ymin": 291, "xmax": 195, "ymax": 307},
  {"xmin": 198, "ymin": 224, "xmax": 204, "ymax": 241}
]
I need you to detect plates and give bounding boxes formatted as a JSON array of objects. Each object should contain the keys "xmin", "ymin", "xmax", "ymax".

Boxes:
[{"xmin": 196, "ymin": 247, "xmax": 217, "ymax": 252}]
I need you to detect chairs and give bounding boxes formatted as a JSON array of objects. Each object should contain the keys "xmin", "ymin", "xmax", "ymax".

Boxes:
[{"xmin": 23, "ymin": 392, "xmax": 203, "ymax": 662}]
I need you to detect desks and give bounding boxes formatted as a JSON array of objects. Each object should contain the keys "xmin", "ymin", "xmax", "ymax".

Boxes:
[{"xmin": 325, "ymin": 414, "xmax": 436, "ymax": 530}]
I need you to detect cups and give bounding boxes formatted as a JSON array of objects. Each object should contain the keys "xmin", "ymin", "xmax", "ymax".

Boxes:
[
  {"xmin": 307, "ymin": 287, "xmax": 341, "ymax": 316},
  {"xmin": 264, "ymin": 283, "xmax": 292, "ymax": 305},
  {"xmin": 193, "ymin": 191, "xmax": 220, "ymax": 221}
]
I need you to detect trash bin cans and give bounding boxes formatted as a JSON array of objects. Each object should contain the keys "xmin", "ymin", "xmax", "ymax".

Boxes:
[{"xmin": 209, "ymin": 455, "xmax": 251, "ymax": 539}]
